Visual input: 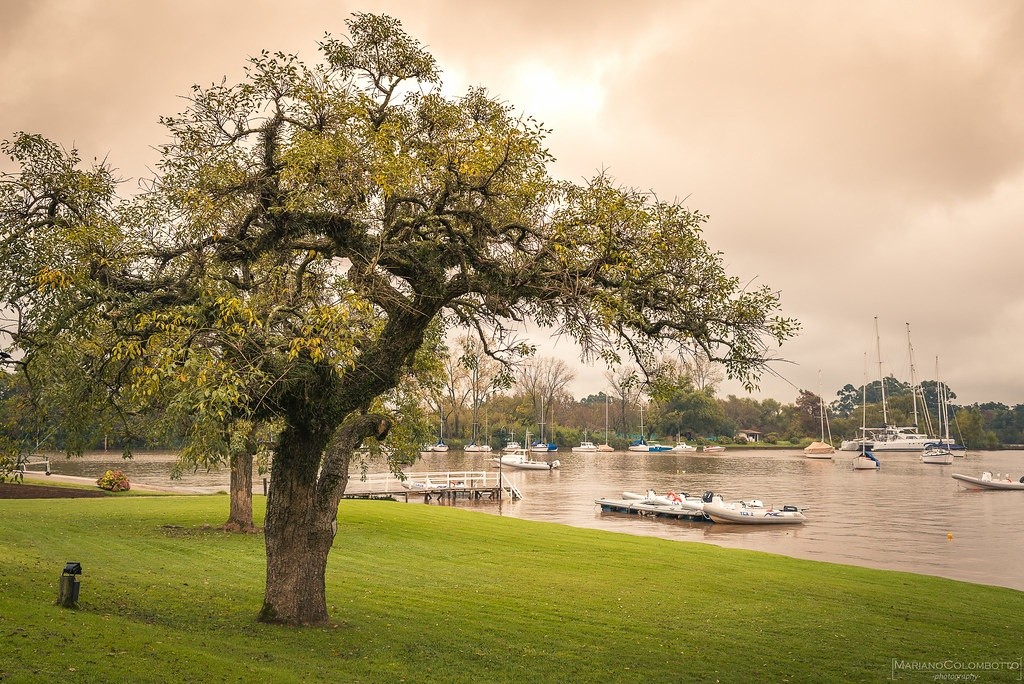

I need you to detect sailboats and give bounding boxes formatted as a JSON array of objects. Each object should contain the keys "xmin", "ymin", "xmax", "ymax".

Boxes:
[
  {"xmin": 838, "ymin": 314, "xmax": 967, "ymax": 469},
  {"xmin": 628, "ymin": 403, "xmax": 673, "ymax": 452},
  {"xmin": 803, "ymin": 369, "xmax": 837, "ymax": 459},
  {"xmin": 424, "ymin": 393, "xmax": 559, "ymax": 453},
  {"xmin": 595, "ymin": 389, "xmax": 615, "ymax": 452}
]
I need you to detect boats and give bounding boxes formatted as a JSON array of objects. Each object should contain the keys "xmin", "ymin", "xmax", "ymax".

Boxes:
[
  {"xmin": 595, "ymin": 489, "xmax": 807, "ymax": 524},
  {"xmin": 671, "ymin": 432, "xmax": 698, "ymax": 451},
  {"xmin": 950, "ymin": 471, "xmax": 1024, "ymax": 490},
  {"xmin": 492, "ymin": 426, "xmax": 560, "ymax": 470},
  {"xmin": 703, "ymin": 445, "xmax": 726, "ymax": 452},
  {"xmin": 570, "ymin": 427, "xmax": 597, "ymax": 452}
]
